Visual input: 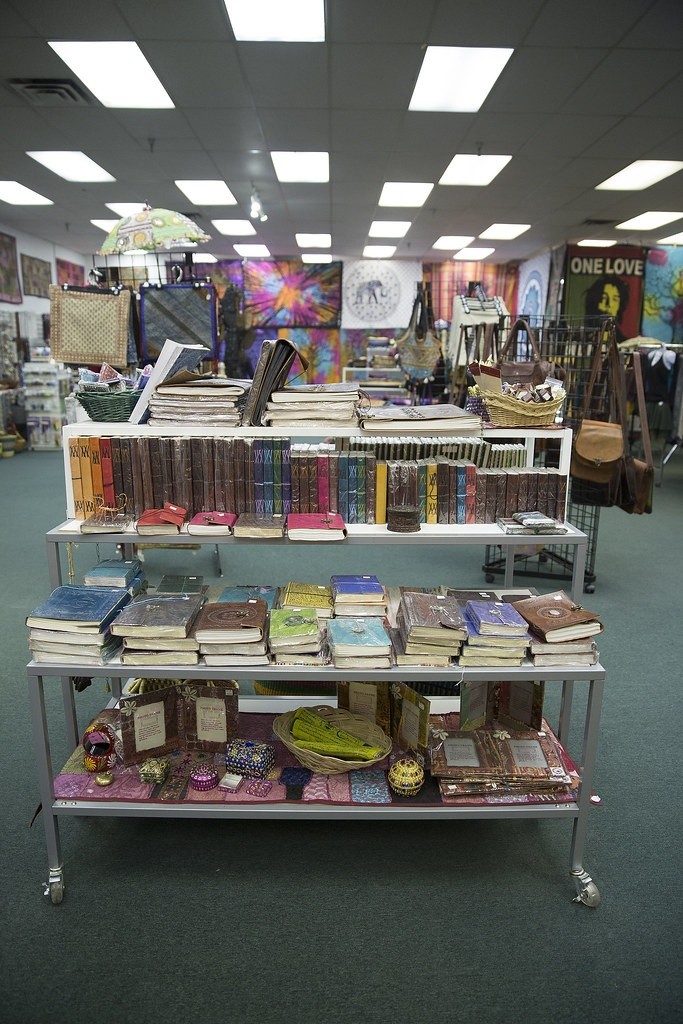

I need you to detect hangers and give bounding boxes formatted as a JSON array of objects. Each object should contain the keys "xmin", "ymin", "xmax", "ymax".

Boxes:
[
  {"xmin": 156, "ymin": 264, "xmax": 211, "ymax": 290},
  {"xmin": 62, "ymin": 270, "xmax": 119, "ymax": 296},
  {"xmin": 461, "ymin": 285, "xmax": 503, "ymax": 316}
]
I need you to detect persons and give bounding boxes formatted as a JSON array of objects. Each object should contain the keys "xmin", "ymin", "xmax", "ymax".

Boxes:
[{"xmin": 583, "ymin": 274, "xmax": 630, "ymax": 342}]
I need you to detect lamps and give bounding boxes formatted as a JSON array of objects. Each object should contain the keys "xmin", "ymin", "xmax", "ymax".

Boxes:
[{"xmin": 252, "ymin": 186, "xmax": 268, "ymax": 221}]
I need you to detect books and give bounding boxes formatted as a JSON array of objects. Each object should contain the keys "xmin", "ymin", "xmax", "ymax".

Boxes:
[{"xmin": 26, "ymin": 339, "xmax": 600, "ymax": 668}]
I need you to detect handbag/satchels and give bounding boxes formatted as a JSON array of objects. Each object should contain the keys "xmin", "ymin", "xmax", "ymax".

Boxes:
[
  {"xmin": 449, "ymin": 319, "xmax": 546, "ymax": 421},
  {"xmin": 396, "ymin": 281, "xmax": 447, "ymax": 398},
  {"xmin": 570, "ymin": 319, "xmax": 654, "ymax": 516},
  {"xmin": 370, "ymin": 355, "xmax": 397, "ymax": 368}
]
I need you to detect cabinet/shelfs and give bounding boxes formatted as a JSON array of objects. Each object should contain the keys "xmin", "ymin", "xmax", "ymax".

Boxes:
[{"xmin": 27, "ymin": 518, "xmax": 606, "ymax": 906}]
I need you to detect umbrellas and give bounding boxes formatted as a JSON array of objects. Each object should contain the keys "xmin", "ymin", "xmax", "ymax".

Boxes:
[{"xmin": 96, "ymin": 198, "xmax": 213, "ymax": 284}]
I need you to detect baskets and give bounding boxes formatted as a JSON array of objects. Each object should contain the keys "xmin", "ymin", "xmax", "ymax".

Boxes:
[
  {"xmin": 273, "ymin": 704, "xmax": 393, "ymax": 774},
  {"xmin": 479, "ymin": 389, "xmax": 566, "ymax": 426},
  {"xmin": 0, "ymin": 430, "xmax": 25, "ymax": 457},
  {"xmin": 74, "ymin": 389, "xmax": 151, "ymax": 424}
]
